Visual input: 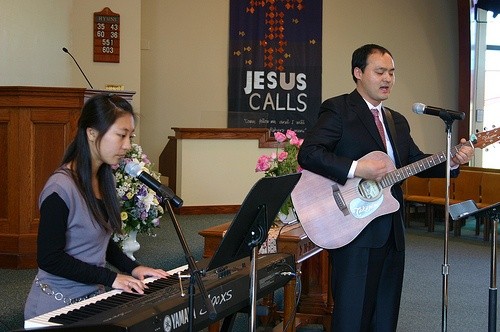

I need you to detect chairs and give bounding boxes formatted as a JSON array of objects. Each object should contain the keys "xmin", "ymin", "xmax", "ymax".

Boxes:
[{"xmin": 402, "ymin": 170, "xmax": 500, "ymax": 240}]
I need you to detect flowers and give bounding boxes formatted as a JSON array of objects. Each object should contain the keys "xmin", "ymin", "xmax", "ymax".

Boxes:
[
  {"xmin": 254, "ymin": 130, "xmax": 305, "ymax": 213},
  {"xmin": 113, "ymin": 143, "xmax": 165, "ymax": 238}
]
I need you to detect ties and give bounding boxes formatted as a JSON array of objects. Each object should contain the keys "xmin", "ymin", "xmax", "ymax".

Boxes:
[{"xmin": 370, "ymin": 109, "xmax": 388, "ymax": 154}]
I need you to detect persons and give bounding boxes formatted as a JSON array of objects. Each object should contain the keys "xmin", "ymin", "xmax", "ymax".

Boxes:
[
  {"xmin": 24, "ymin": 95, "xmax": 170, "ymax": 320},
  {"xmin": 298, "ymin": 44, "xmax": 475, "ymax": 332}
]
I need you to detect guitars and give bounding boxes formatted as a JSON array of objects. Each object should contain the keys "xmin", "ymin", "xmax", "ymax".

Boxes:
[{"xmin": 290, "ymin": 123, "xmax": 500, "ymax": 251}]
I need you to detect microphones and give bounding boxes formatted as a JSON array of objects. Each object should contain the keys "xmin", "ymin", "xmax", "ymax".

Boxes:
[
  {"xmin": 62, "ymin": 48, "xmax": 94, "ymax": 89},
  {"xmin": 413, "ymin": 102, "xmax": 465, "ymax": 121},
  {"xmin": 125, "ymin": 162, "xmax": 183, "ymax": 208}
]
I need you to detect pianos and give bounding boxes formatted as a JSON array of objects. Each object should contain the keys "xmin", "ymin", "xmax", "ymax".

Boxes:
[{"xmin": 24, "ymin": 253, "xmax": 297, "ymax": 332}]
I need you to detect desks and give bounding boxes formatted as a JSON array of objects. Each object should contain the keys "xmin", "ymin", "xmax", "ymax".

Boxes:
[
  {"xmin": 197, "ymin": 220, "xmax": 330, "ymax": 332},
  {"xmin": 168, "ymin": 127, "xmax": 289, "ymax": 213}
]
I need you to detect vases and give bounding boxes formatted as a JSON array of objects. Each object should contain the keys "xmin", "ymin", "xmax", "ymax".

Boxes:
[{"xmin": 122, "ymin": 231, "xmax": 140, "ymax": 261}]
